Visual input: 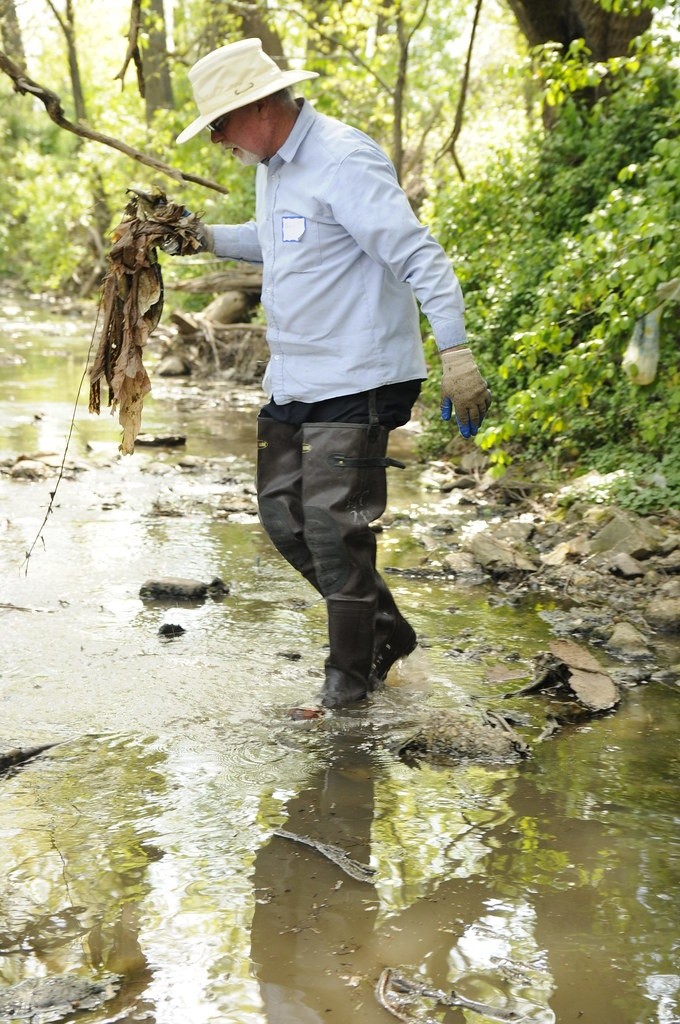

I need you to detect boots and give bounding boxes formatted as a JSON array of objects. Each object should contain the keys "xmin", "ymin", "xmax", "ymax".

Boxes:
[
  {"xmin": 322, "ymin": 600, "xmax": 379, "ymax": 711},
  {"xmin": 357, "ymin": 582, "xmax": 418, "ymax": 692}
]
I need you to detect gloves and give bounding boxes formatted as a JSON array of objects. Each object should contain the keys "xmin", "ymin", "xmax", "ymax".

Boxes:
[
  {"xmin": 160, "ymin": 205, "xmax": 213, "ymax": 256},
  {"xmin": 440, "ymin": 348, "xmax": 492, "ymax": 438}
]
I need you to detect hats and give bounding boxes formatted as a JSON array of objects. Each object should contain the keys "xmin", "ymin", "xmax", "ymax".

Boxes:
[{"xmin": 175, "ymin": 38, "xmax": 320, "ymax": 146}]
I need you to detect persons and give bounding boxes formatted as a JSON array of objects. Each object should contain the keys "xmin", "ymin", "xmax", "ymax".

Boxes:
[{"xmin": 153, "ymin": 37, "xmax": 492, "ymax": 718}]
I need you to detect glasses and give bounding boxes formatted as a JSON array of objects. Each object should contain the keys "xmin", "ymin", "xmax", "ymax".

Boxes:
[{"xmin": 206, "ymin": 113, "xmax": 229, "ymax": 132}]
